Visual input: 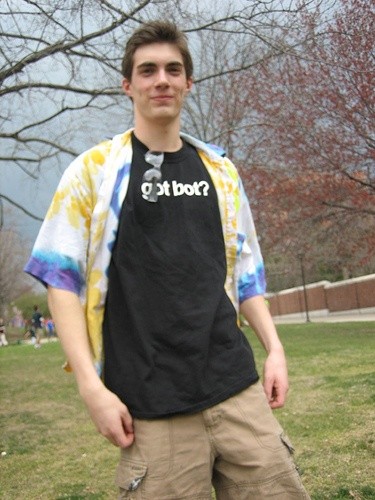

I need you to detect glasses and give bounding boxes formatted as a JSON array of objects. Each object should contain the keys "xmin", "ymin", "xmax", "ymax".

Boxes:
[{"xmin": 142, "ymin": 150, "xmax": 164, "ymax": 184}]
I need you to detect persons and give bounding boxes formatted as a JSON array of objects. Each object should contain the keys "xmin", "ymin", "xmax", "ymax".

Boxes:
[
  {"xmin": 23, "ymin": 20, "xmax": 311, "ymax": 500},
  {"xmin": 0, "ymin": 305, "xmax": 53, "ymax": 349}
]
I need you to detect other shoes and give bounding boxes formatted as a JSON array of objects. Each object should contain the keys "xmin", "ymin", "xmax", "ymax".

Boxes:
[{"xmin": 34, "ymin": 343, "xmax": 40, "ymax": 349}]
